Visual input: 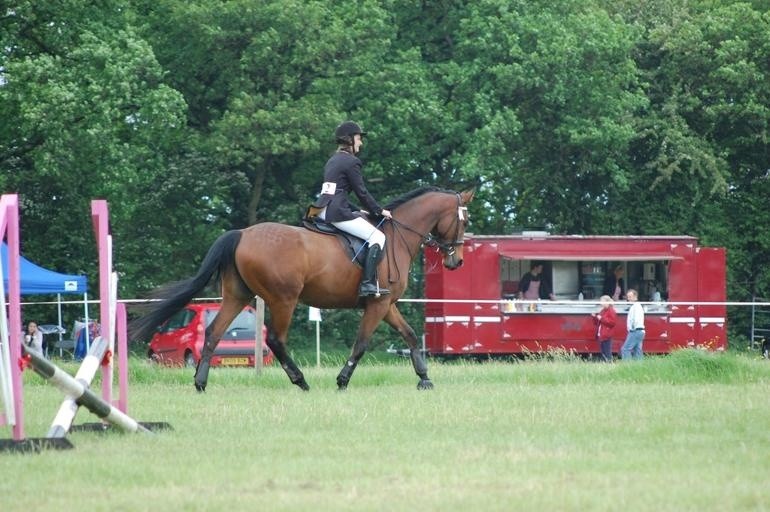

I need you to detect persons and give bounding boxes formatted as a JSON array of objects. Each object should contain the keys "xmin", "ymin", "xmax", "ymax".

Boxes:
[
  {"xmin": 603, "ymin": 264, "xmax": 628, "ymax": 300},
  {"xmin": 620, "ymin": 288, "xmax": 647, "ymax": 360},
  {"xmin": 591, "ymin": 295, "xmax": 617, "ymax": 362},
  {"xmin": 22, "ymin": 318, "xmax": 44, "ymax": 360},
  {"xmin": 514, "ymin": 261, "xmax": 559, "ymax": 301},
  {"xmin": 304, "ymin": 119, "xmax": 394, "ymax": 298}
]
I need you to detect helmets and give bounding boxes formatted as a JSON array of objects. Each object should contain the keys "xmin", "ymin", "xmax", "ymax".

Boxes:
[{"xmin": 336, "ymin": 121, "xmax": 367, "ymax": 138}]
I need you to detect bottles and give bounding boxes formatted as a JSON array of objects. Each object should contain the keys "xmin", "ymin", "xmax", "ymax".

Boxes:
[
  {"xmin": 579, "ymin": 292, "xmax": 584, "ymax": 303},
  {"xmin": 500, "ymin": 297, "xmax": 544, "ymax": 314}
]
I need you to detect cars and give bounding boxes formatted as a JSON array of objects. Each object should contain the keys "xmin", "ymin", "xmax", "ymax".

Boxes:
[{"xmin": 147, "ymin": 303, "xmax": 273, "ymax": 368}]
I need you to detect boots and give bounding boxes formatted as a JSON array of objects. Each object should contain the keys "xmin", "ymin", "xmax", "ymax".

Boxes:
[{"xmin": 358, "ymin": 243, "xmax": 391, "ymax": 297}]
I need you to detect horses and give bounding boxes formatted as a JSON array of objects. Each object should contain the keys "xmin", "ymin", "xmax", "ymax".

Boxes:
[{"xmin": 124, "ymin": 185, "xmax": 477, "ymax": 392}]
